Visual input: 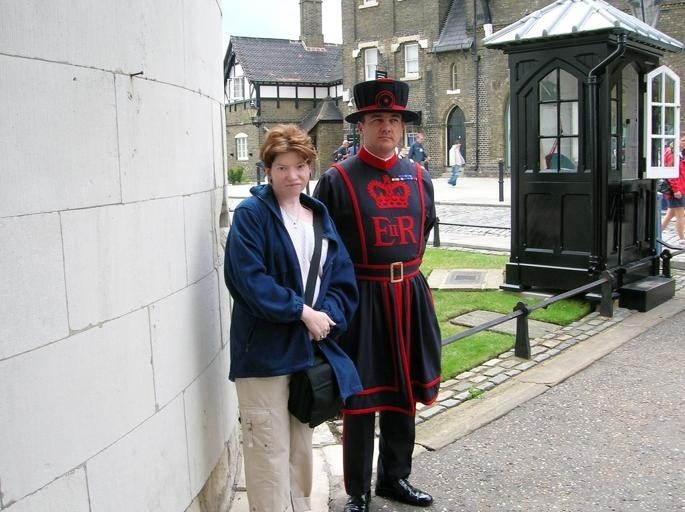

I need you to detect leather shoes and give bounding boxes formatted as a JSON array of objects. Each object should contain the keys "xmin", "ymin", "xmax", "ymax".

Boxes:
[
  {"xmin": 346, "ymin": 490, "xmax": 371, "ymax": 511},
  {"xmin": 375, "ymin": 477, "xmax": 433, "ymax": 507}
]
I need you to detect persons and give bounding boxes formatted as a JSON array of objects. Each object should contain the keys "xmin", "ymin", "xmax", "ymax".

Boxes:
[
  {"xmin": 659, "ymin": 130, "xmax": 685, "ymax": 245},
  {"xmin": 337, "ymin": 139, "xmax": 352, "ymax": 161},
  {"xmin": 446, "ymin": 139, "xmax": 466, "ymax": 188},
  {"xmin": 408, "ymin": 133, "xmax": 431, "ymax": 169},
  {"xmin": 222, "ymin": 121, "xmax": 360, "ymax": 511},
  {"xmin": 347, "ymin": 141, "xmax": 359, "ymax": 157},
  {"xmin": 310, "ymin": 78, "xmax": 449, "ymax": 511}
]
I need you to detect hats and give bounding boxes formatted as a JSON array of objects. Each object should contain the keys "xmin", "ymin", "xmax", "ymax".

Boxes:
[{"xmin": 345, "ymin": 79, "xmax": 418, "ymax": 123}]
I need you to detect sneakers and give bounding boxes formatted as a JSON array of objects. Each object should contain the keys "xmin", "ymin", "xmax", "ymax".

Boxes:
[{"xmin": 677, "ymin": 240, "xmax": 685, "ymax": 248}]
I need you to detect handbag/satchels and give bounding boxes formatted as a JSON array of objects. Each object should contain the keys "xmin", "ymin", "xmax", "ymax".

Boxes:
[
  {"xmin": 287, "ymin": 338, "xmax": 342, "ymax": 429},
  {"xmin": 657, "ymin": 179, "xmax": 672, "ymax": 194}
]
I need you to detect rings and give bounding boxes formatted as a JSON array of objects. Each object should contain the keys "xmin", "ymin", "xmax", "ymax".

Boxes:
[{"xmin": 320, "ymin": 335, "xmax": 323, "ymax": 339}]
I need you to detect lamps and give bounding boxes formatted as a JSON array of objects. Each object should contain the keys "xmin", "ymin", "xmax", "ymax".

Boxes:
[{"xmin": 247, "ymin": 100, "xmax": 260, "ymax": 128}]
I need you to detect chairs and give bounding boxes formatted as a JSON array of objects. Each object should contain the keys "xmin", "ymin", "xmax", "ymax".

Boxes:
[{"xmin": 546, "ymin": 153, "xmax": 575, "ymax": 169}]
